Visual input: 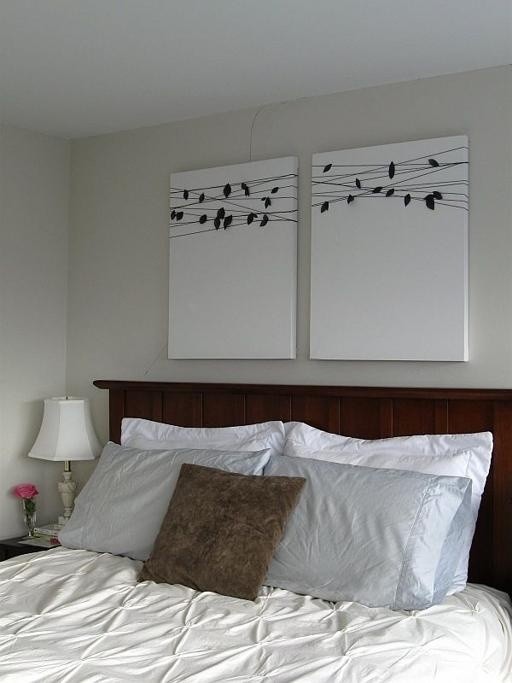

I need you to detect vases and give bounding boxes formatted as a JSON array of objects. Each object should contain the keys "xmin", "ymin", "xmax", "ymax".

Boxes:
[{"xmin": 24, "ymin": 511, "xmax": 40, "ymax": 539}]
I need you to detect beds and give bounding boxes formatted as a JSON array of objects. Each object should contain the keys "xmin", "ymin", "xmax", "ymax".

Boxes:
[{"xmin": 1, "ymin": 378, "xmax": 512, "ymax": 683}]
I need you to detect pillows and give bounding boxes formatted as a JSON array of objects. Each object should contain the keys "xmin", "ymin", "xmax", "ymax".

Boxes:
[
  {"xmin": 284, "ymin": 419, "xmax": 493, "ymax": 599},
  {"xmin": 262, "ymin": 456, "xmax": 472, "ymax": 611},
  {"xmin": 136, "ymin": 462, "xmax": 308, "ymax": 604},
  {"xmin": 116, "ymin": 418, "xmax": 284, "ymax": 455},
  {"xmin": 59, "ymin": 441, "xmax": 273, "ymax": 562}
]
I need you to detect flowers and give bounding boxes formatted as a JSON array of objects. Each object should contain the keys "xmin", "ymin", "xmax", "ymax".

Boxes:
[{"xmin": 13, "ymin": 483, "xmax": 39, "ymax": 529}]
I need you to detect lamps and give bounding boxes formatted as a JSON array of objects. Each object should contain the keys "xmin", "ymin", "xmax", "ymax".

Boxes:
[{"xmin": 28, "ymin": 395, "xmax": 103, "ymax": 530}]
